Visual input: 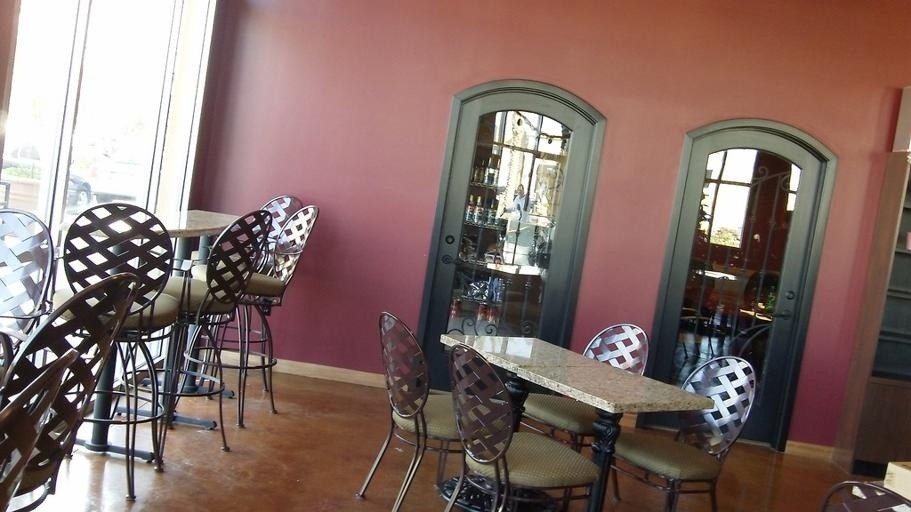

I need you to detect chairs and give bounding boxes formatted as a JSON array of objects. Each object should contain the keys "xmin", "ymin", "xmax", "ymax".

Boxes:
[
  {"xmin": 820, "ymin": 481, "xmax": 911, "ymax": 512},
  {"xmin": 446, "ymin": 342, "xmax": 600, "ymax": 510},
  {"xmin": 359, "ymin": 311, "xmax": 511, "ymax": 510},
  {"xmin": 0, "ymin": 194, "xmax": 322, "ymax": 512},
  {"xmin": 515, "ymin": 323, "xmax": 650, "ymax": 509},
  {"xmin": 586, "ymin": 356, "xmax": 756, "ymax": 511}
]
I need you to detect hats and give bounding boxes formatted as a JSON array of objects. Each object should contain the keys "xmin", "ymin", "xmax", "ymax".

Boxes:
[
  {"xmin": 3, "ymin": 159, "xmax": 90, "ymax": 209},
  {"xmin": 67, "ymin": 161, "xmax": 145, "ymax": 204}
]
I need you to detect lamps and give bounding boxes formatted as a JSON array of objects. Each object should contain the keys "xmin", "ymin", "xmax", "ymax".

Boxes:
[{"xmin": 820, "ymin": 481, "xmax": 911, "ymax": 512}]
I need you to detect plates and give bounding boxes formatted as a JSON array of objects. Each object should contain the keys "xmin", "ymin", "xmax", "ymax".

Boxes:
[{"xmin": 827, "ymin": 152, "xmax": 911, "ymax": 478}]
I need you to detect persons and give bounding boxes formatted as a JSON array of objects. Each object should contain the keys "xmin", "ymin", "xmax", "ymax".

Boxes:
[{"xmin": 504, "ymin": 184, "xmax": 531, "ymax": 224}]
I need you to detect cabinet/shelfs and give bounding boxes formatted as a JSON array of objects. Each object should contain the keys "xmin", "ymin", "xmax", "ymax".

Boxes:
[
  {"xmin": 827, "ymin": 152, "xmax": 911, "ymax": 478},
  {"xmin": 466, "ymin": 194, "xmax": 507, "ymax": 228},
  {"xmin": 473, "ymin": 155, "xmax": 506, "ymax": 186}
]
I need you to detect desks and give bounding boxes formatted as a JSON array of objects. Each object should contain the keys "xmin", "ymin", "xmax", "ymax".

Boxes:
[{"xmin": 440, "ymin": 334, "xmax": 715, "ymax": 510}]
